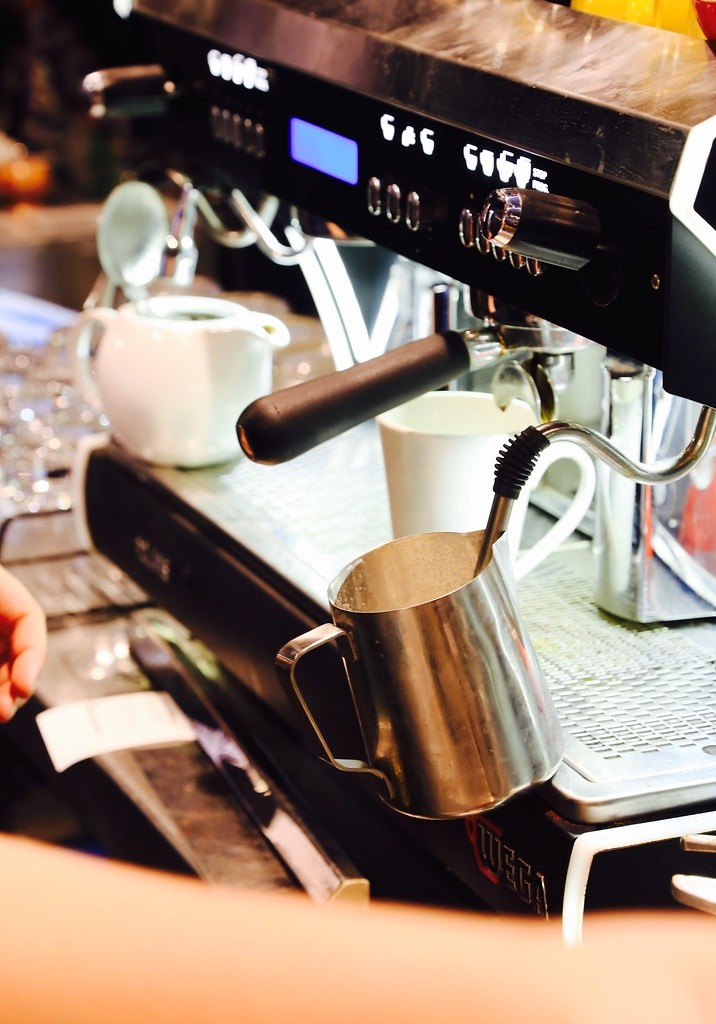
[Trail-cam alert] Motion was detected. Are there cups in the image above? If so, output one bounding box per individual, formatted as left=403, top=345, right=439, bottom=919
left=375, top=390, right=596, bottom=582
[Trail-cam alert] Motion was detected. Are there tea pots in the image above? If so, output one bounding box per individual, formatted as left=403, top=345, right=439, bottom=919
left=275, top=531, right=564, bottom=825
left=67, top=294, right=291, bottom=469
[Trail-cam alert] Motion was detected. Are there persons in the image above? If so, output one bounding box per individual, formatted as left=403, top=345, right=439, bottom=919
left=0, top=564, right=716, bottom=1024
